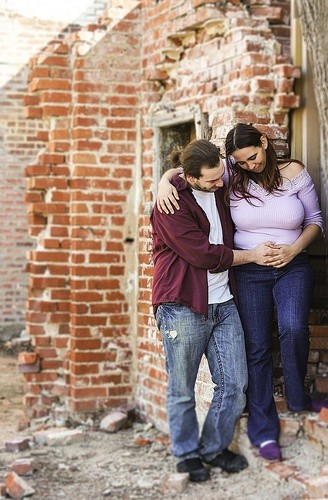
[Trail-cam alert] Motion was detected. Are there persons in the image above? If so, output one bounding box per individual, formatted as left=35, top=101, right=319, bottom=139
left=157, top=122, right=328, bottom=460
left=150, top=137, right=281, bottom=483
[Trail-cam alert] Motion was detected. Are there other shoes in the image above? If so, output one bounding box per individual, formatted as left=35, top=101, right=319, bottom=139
left=176, top=458, right=209, bottom=482
left=259, top=440, right=281, bottom=462
left=211, top=447, right=247, bottom=472
left=309, top=397, right=328, bottom=413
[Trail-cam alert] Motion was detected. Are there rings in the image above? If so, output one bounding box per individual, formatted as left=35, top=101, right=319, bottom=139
left=280, top=260, right=283, bottom=264
